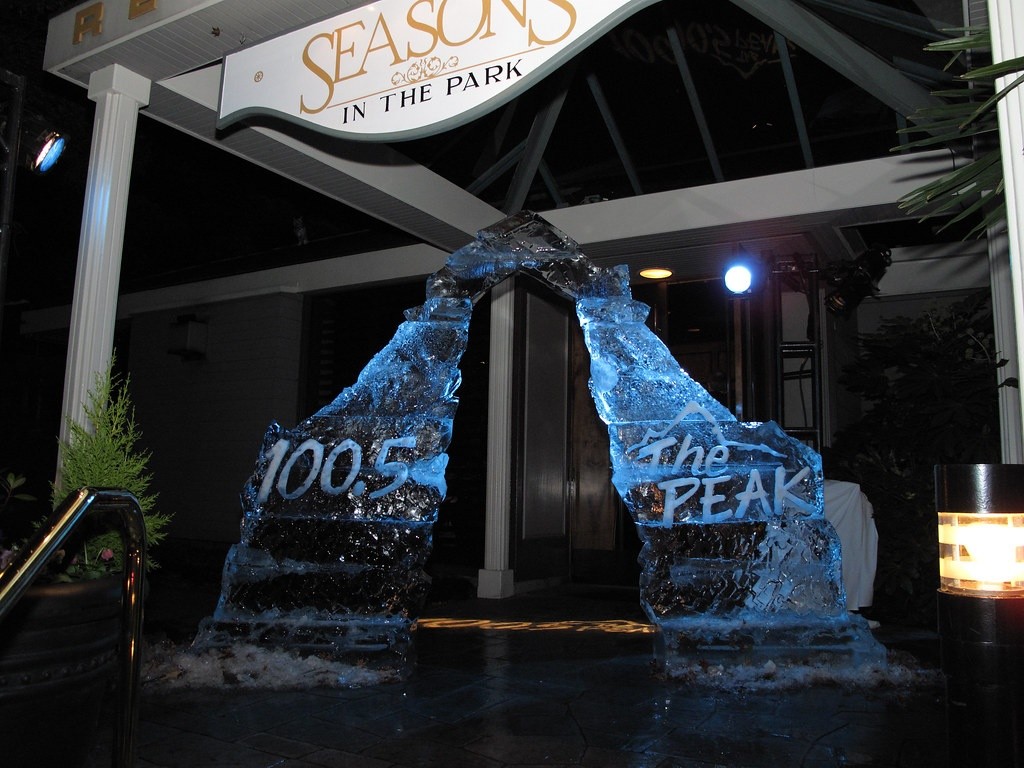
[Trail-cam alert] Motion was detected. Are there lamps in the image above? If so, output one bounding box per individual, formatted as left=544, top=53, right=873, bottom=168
left=828, top=265, right=874, bottom=312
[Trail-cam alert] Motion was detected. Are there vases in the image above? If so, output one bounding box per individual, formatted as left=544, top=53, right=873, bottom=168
left=0, top=574, right=124, bottom=768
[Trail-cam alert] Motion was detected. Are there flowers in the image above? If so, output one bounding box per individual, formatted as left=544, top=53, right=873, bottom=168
left=0, top=347, right=175, bottom=585
